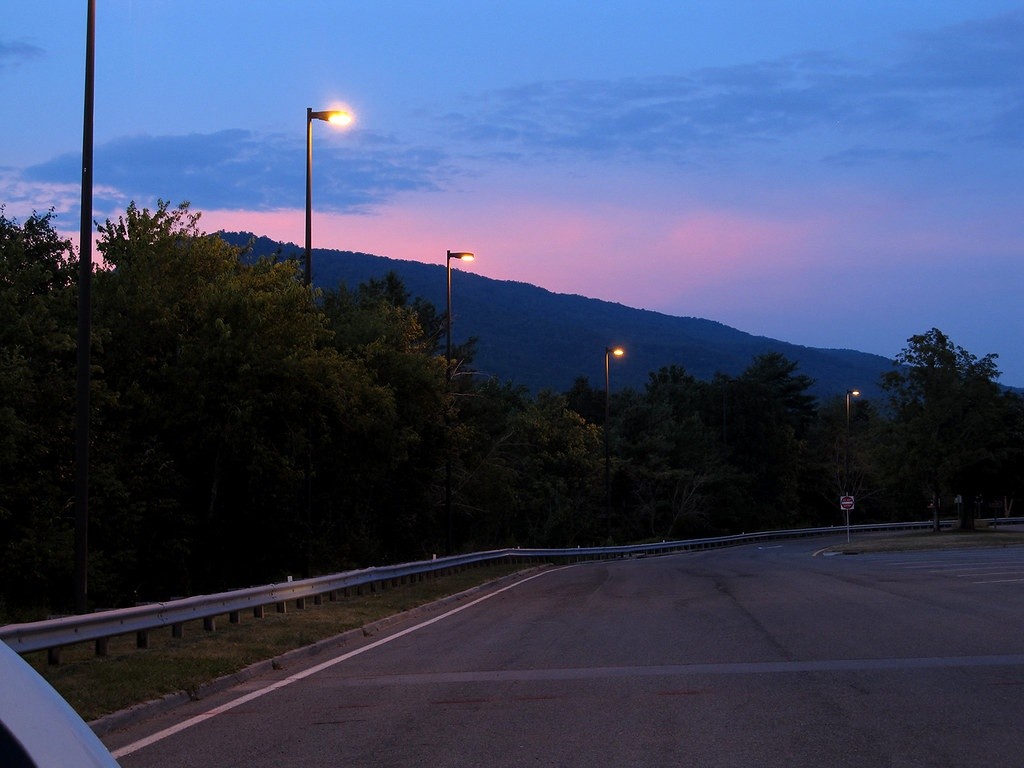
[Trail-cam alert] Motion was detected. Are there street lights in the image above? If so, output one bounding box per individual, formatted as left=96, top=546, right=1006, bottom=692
left=301, top=108, right=365, bottom=560
left=845, top=389, right=860, bottom=496
left=604, top=345, right=625, bottom=547
left=445, top=250, right=477, bottom=556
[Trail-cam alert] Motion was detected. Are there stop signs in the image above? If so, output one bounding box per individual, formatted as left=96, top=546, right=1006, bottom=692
left=840, top=495, right=855, bottom=511
